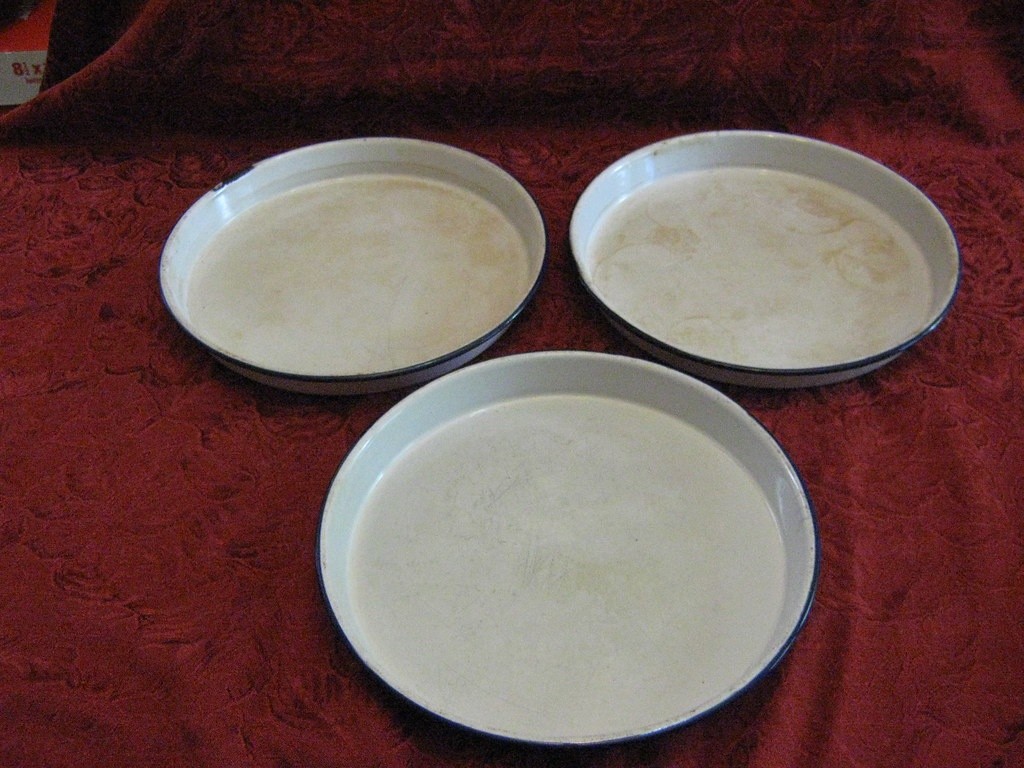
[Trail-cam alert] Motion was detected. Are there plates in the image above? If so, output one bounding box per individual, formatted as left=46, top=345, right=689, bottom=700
left=161, top=134, right=548, bottom=396
left=315, top=351, right=818, bottom=746
left=569, top=129, right=960, bottom=389
